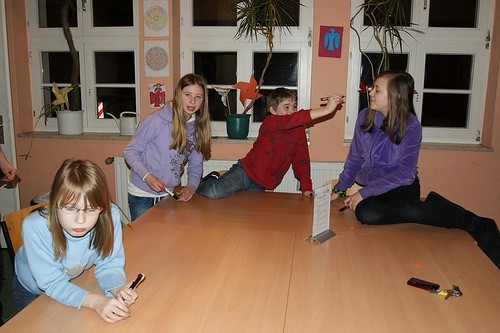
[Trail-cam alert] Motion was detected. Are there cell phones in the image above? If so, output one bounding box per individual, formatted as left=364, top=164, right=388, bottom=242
left=407, top=278, right=440, bottom=291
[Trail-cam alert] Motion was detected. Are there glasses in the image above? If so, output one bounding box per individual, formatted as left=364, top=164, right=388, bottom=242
left=55, top=203, right=102, bottom=216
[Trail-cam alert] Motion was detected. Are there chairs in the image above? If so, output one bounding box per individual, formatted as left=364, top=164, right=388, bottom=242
left=2, top=201, right=52, bottom=267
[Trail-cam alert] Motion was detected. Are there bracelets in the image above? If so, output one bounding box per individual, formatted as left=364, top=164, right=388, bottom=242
left=332, top=187, right=347, bottom=197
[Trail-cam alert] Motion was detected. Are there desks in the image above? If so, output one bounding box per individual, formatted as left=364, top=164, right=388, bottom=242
left=0, top=187, right=500, bottom=333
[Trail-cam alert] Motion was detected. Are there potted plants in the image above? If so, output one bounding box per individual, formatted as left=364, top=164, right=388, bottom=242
left=224, top=0, right=309, bottom=140
left=19, top=0, right=84, bottom=162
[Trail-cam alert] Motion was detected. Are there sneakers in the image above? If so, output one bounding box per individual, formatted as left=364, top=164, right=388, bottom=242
left=200, top=171, right=221, bottom=180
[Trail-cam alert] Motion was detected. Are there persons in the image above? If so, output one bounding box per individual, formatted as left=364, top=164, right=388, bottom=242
left=329, top=70, right=497, bottom=243
left=196, top=88, right=345, bottom=200
left=123, top=73, right=212, bottom=222
left=13, top=158, right=138, bottom=324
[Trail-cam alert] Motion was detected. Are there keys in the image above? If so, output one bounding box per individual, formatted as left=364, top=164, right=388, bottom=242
left=444, top=290, right=454, bottom=300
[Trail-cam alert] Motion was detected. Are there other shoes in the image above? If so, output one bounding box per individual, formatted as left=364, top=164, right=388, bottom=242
left=472, top=215, right=500, bottom=266
left=425, top=190, right=465, bottom=209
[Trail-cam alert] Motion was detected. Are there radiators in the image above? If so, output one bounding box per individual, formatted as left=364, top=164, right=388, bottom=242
left=103, top=156, right=346, bottom=225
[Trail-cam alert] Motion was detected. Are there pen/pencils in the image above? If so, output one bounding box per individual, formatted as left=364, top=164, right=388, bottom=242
left=339, top=205, right=350, bottom=212
left=164, top=188, right=178, bottom=200
left=321, top=96, right=346, bottom=101
left=122, top=273, right=145, bottom=301
